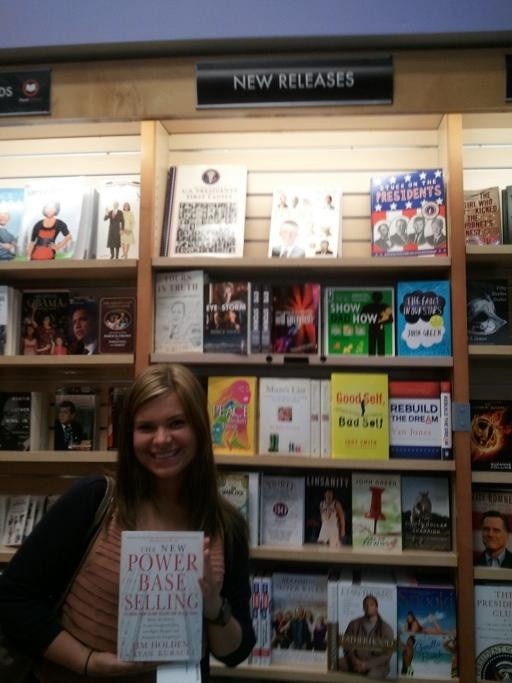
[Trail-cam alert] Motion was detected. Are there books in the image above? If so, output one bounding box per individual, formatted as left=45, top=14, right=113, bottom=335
left=2, top=174, right=146, bottom=563
left=151, top=267, right=512, bottom=683
left=118, top=530, right=204, bottom=663
left=152, top=157, right=512, bottom=259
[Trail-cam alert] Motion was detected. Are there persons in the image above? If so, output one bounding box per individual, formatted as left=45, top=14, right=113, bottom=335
left=2, top=360, right=257, bottom=683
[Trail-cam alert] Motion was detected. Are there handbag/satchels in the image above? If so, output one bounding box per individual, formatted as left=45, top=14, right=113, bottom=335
left=0, top=637, right=32, bottom=681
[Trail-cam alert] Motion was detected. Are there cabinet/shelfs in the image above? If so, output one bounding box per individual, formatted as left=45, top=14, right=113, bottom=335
left=0, top=112, right=511, bottom=680
